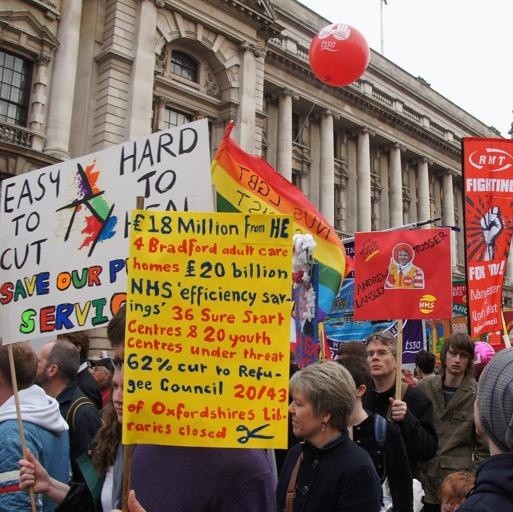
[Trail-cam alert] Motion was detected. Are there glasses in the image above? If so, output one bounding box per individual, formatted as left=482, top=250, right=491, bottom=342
left=367, top=350, right=397, bottom=356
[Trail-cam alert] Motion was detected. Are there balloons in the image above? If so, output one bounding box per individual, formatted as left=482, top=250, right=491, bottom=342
left=307, top=21, right=371, bottom=88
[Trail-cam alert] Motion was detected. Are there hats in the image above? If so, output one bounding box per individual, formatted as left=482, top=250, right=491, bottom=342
left=89, top=358, right=115, bottom=373
left=476, top=346, right=513, bottom=455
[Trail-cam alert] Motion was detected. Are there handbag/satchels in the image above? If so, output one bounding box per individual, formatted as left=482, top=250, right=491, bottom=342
left=380, top=472, right=426, bottom=511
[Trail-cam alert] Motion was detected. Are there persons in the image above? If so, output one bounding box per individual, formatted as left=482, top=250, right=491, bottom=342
left=479, top=205, right=505, bottom=261
left=381, top=241, right=425, bottom=290
left=2, top=302, right=513, bottom=511
